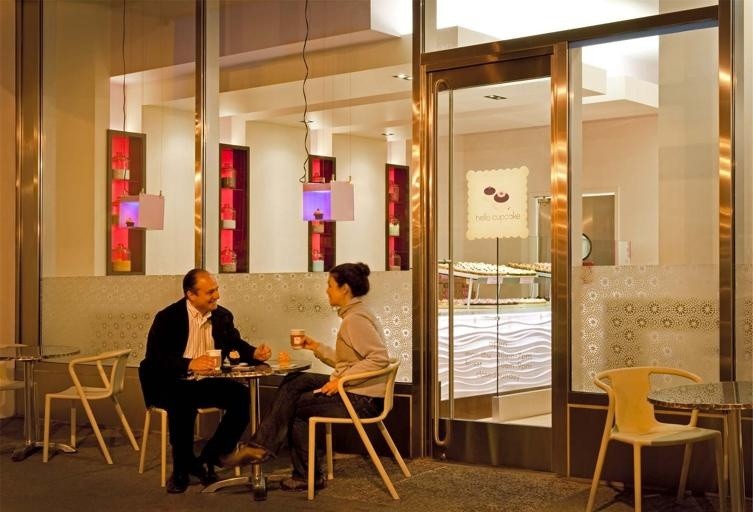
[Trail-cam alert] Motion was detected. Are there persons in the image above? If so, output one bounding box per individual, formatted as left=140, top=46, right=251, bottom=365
left=138, top=267, right=272, bottom=495
left=221, top=260, right=389, bottom=491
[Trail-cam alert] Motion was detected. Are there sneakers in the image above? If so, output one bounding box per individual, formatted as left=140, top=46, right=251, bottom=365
left=279, top=475, right=324, bottom=491
left=166, top=468, right=190, bottom=494
left=190, top=455, right=220, bottom=484
left=221, top=440, right=272, bottom=466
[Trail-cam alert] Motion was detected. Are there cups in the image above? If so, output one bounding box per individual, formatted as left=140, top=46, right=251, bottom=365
left=205, top=349, right=222, bottom=372
left=290, top=328, right=305, bottom=350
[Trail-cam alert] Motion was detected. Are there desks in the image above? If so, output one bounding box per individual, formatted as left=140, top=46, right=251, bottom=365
left=647, top=380, right=753, bottom=512
left=0, top=345, right=80, bottom=462
left=195, top=359, right=312, bottom=500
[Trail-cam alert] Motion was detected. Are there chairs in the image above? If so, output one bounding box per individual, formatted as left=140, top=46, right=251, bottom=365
left=138, top=408, right=241, bottom=487
left=43, top=349, right=140, bottom=464
left=0, top=344, right=39, bottom=440
left=586, top=367, right=727, bottom=512
left=308, top=358, right=412, bottom=499
